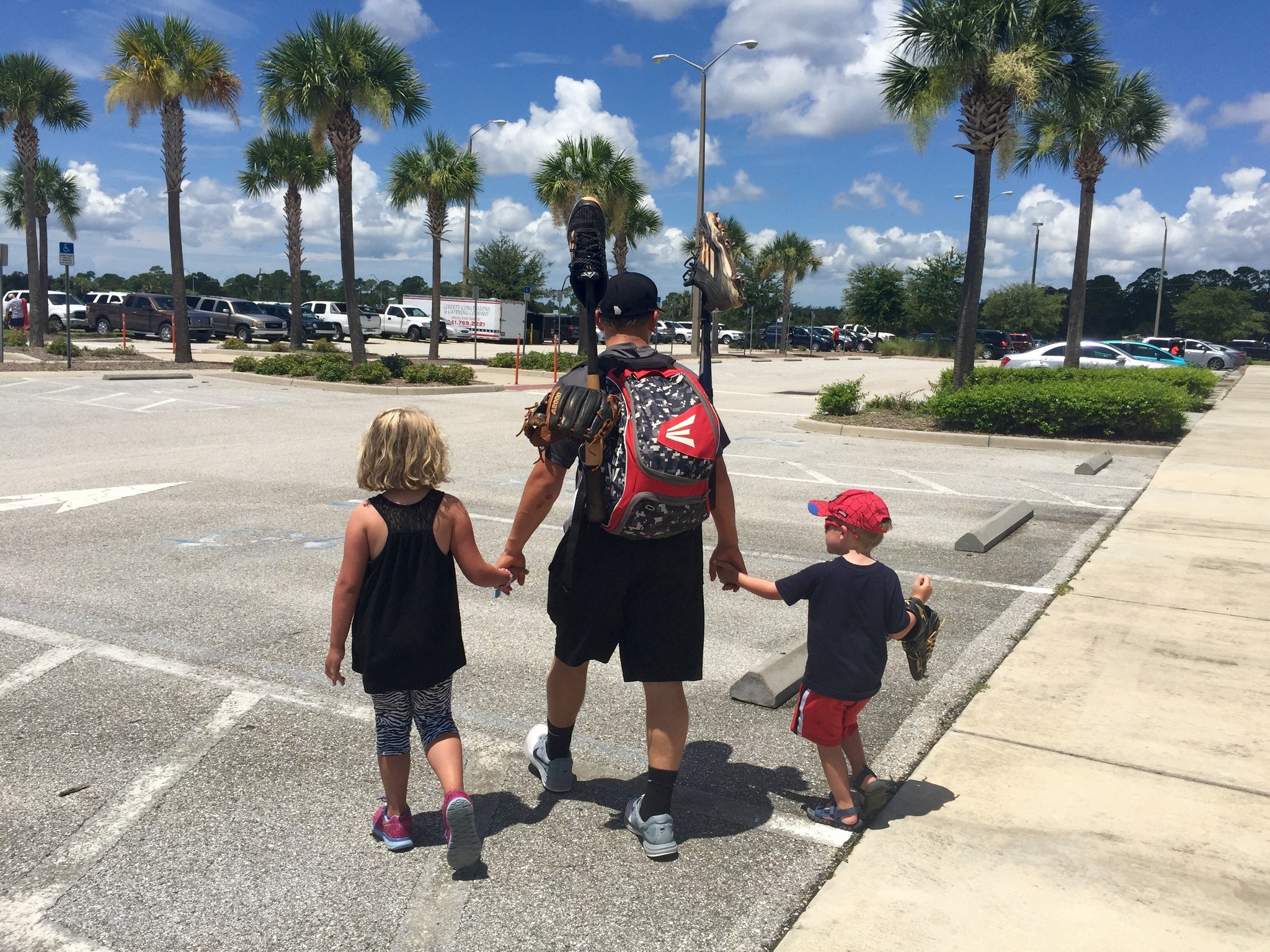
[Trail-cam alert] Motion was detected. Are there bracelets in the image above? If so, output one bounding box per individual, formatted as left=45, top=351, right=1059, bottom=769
left=494, top=568, right=513, bottom=598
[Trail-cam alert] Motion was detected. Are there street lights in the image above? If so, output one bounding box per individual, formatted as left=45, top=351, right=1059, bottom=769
left=184, top=267, right=194, bottom=294
left=1153, top=215, right=1169, bottom=337
left=369, top=273, right=383, bottom=306
left=459, top=119, right=507, bottom=297
left=1032, top=220, right=1043, bottom=283
left=653, top=40, right=755, bottom=353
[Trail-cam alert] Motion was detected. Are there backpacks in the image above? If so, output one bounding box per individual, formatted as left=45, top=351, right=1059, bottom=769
left=572, top=352, right=720, bottom=539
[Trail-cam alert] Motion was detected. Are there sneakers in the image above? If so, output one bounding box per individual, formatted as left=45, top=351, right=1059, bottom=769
left=523, top=723, right=573, bottom=792
left=441, top=788, right=481, bottom=868
left=371, top=796, right=413, bottom=851
left=623, top=794, right=678, bottom=858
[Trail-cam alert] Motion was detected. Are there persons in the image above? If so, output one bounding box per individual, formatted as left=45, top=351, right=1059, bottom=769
left=832, top=327, right=840, bottom=352
left=324, top=408, right=529, bottom=868
left=714, top=489, right=933, bottom=832
left=498, top=271, right=748, bottom=857
left=1171, top=343, right=1179, bottom=355
left=5, top=294, right=24, bottom=335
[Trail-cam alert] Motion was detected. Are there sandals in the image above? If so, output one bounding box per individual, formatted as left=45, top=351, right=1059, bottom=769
left=806, top=796, right=863, bottom=830
left=853, top=765, right=888, bottom=813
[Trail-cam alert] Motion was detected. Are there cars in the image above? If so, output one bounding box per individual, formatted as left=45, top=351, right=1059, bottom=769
left=1001, top=340, right=1181, bottom=369
left=1084, top=341, right=1202, bottom=369
left=539, top=312, right=1047, bottom=358
left=429, top=315, right=472, bottom=342
left=1202, top=340, right=1270, bottom=366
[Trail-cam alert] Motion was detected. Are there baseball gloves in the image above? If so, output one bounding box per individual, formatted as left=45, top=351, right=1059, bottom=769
left=901, top=596, right=940, bottom=681
left=524, top=383, right=618, bottom=449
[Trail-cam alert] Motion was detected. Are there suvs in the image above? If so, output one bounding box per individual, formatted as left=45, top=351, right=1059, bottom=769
left=84, top=291, right=337, bottom=343
left=1143, top=336, right=1234, bottom=370
left=2, top=289, right=96, bottom=333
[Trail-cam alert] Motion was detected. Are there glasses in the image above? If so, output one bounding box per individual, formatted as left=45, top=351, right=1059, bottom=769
left=825, top=518, right=858, bottom=539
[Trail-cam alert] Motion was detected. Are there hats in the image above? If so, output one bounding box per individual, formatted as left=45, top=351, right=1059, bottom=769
left=598, top=272, right=665, bottom=317
left=808, top=489, right=891, bottom=533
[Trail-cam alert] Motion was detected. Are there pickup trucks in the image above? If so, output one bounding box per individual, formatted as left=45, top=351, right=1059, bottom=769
left=86, top=294, right=214, bottom=343
left=301, top=302, right=382, bottom=343
left=377, top=304, right=447, bottom=343
